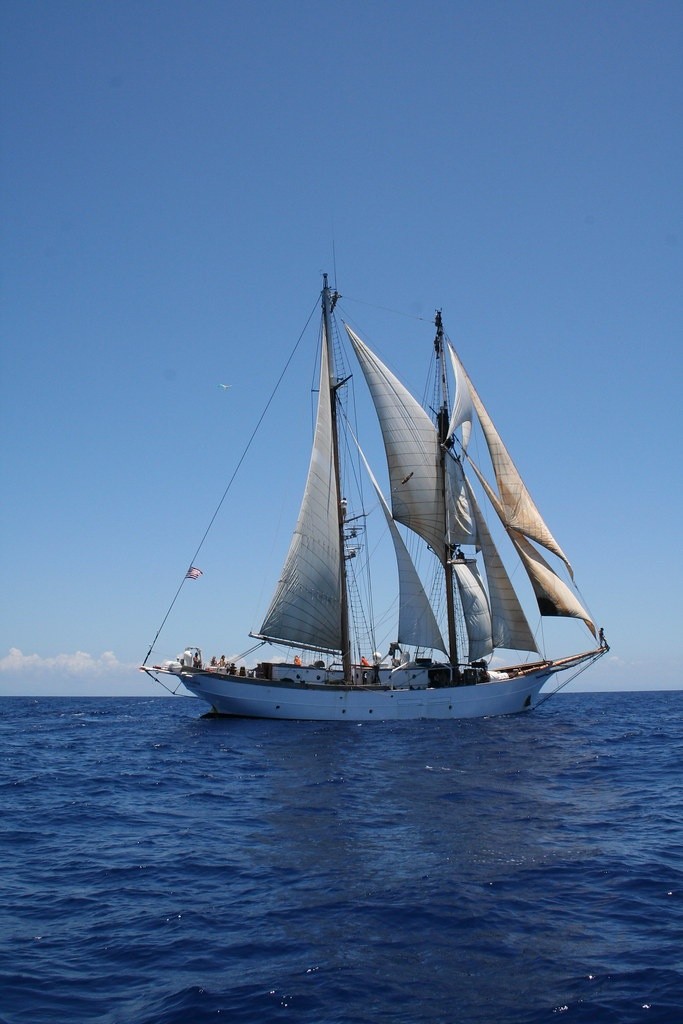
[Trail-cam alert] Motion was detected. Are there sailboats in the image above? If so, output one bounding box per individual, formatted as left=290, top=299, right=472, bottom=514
left=138, top=270, right=615, bottom=725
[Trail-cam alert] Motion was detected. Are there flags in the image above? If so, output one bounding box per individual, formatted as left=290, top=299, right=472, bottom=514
left=185, top=567, right=203, bottom=580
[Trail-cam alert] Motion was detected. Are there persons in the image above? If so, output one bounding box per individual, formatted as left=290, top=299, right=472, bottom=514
left=362, top=656, right=369, bottom=666
left=339, top=498, right=347, bottom=522
left=294, top=655, right=302, bottom=665
left=220, top=655, right=230, bottom=668
left=211, top=656, right=216, bottom=666
left=457, top=549, right=464, bottom=559
left=193, top=652, right=198, bottom=667
